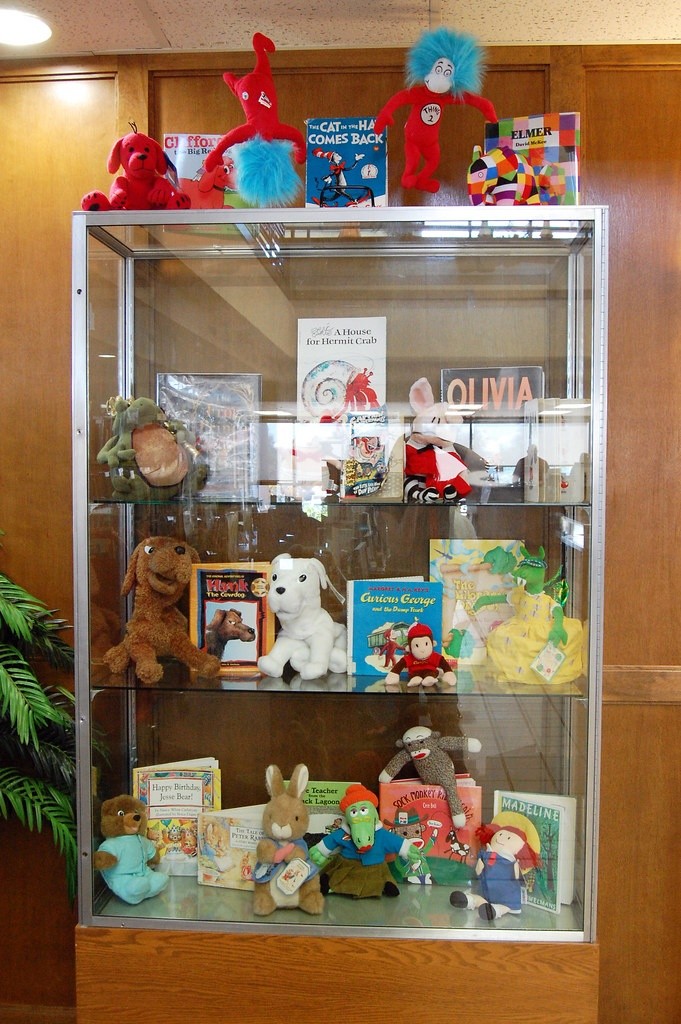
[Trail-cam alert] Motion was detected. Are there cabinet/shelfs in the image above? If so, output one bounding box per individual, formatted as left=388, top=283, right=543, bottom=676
left=68, top=203, right=600, bottom=1024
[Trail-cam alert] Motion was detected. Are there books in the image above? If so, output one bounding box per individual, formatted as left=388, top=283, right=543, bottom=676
left=163, top=108, right=581, bottom=238
left=186, top=539, right=526, bottom=671
left=131, top=755, right=579, bottom=913
left=155, top=316, right=585, bottom=504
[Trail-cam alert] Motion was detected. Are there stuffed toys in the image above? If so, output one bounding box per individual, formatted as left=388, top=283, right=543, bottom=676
left=97, top=394, right=209, bottom=500
left=379, top=727, right=482, bottom=828
left=103, top=537, right=220, bottom=681
left=385, top=620, right=458, bottom=686
left=251, top=762, right=324, bottom=917
left=257, top=553, right=347, bottom=679
left=402, top=377, right=474, bottom=499
left=93, top=795, right=170, bottom=906
left=203, top=33, right=305, bottom=207
left=472, top=545, right=583, bottom=685
left=81, top=122, right=192, bottom=211
left=373, top=25, right=500, bottom=193
left=308, top=785, right=423, bottom=897
left=449, top=809, right=542, bottom=920
